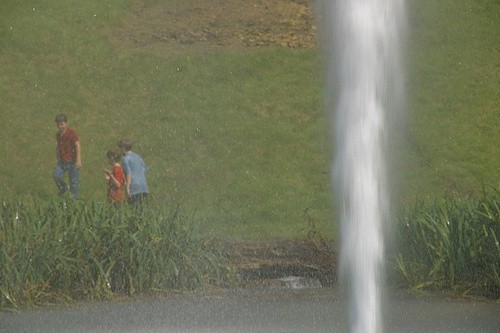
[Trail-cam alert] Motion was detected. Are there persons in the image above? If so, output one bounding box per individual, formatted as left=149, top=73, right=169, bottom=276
left=103, top=148, right=125, bottom=204
left=118, top=139, right=150, bottom=205
left=53, top=113, right=82, bottom=197
